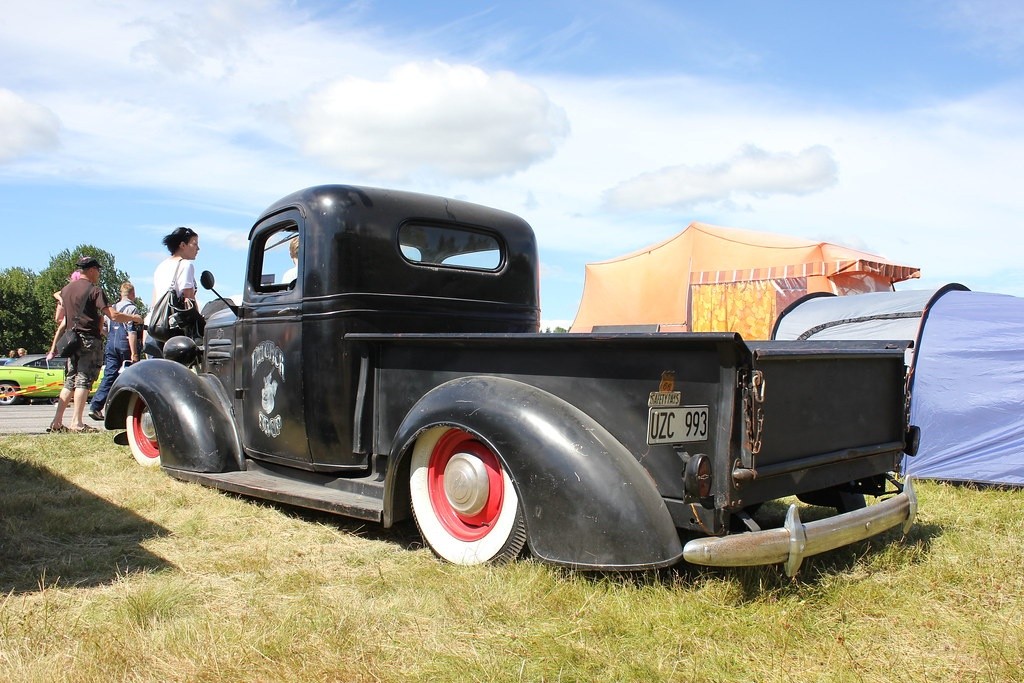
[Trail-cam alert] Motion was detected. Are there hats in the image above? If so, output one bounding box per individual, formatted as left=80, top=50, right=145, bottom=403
left=76, top=256, right=104, bottom=269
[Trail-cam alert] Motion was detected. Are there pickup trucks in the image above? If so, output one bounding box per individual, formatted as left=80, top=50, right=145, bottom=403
left=103, top=184, right=923, bottom=578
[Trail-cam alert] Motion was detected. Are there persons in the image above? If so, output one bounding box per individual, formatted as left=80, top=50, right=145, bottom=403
left=147, top=227, right=201, bottom=367
left=47, top=257, right=144, bottom=433
left=18, top=348, right=25, bottom=357
left=10, top=350, right=17, bottom=358
left=283, top=236, right=299, bottom=283
left=89, top=283, right=140, bottom=420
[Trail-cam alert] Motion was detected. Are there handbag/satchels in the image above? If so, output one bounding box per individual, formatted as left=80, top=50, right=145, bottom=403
left=56, top=330, right=83, bottom=358
left=147, top=258, right=196, bottom=342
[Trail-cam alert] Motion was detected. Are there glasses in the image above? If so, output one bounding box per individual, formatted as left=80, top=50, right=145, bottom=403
left=182, top=229, right=193, bottom=241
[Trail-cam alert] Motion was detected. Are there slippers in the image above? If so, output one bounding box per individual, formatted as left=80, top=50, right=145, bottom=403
left=70, top=424, right=98, bottom=433
left=46, top=425, right=69, bottom=433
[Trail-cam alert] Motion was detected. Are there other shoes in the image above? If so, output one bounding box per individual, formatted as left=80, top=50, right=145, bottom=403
left=89, top=409, right=105, bottom=421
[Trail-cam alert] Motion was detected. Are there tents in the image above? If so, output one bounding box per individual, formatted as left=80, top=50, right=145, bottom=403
left=569, top=222, right=921, bottom=343
left=771, top=282, right=1024, bottom=486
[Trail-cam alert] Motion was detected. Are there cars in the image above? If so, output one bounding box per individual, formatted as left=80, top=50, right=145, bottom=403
left=0, top=353, right=106, bottom=406
